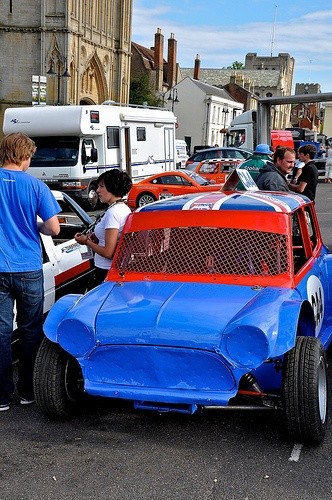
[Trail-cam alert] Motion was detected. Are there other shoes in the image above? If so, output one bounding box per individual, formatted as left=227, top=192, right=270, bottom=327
left=0, top=396, right=15, bottom=411
left=20, top=394, right=36, bottom=404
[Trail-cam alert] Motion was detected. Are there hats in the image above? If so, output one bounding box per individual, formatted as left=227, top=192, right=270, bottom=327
left=253, top=144, right=272, bottom=154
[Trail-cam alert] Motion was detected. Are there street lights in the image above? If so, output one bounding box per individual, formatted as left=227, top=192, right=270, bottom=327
left=222, top=102, right=230, bottom=128
left=168, top=84, right=180, bottom=111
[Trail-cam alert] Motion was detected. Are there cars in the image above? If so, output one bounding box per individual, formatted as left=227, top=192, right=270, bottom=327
left=175, top=140, right=254, bottom=183
left=127, top=170, right=224, bottom=207
left=13, top=190, right=97, bottom=329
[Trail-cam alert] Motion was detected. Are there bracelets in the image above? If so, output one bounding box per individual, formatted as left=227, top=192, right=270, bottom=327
left=83, top=238, right=90, bottom=245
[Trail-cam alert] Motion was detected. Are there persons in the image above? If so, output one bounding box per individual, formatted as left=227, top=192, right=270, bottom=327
left=255, top=147, right=296, bottom=192
left=320, top=136, right=332, bottom=184
left=268, top=145, right=274, bottom=158
left=0, top=131, right=64, bottom=412
left=291, top=145, right=305, bottom=184
left=235, top=143, right=276, bottom=191
left=72, top=168, right=133, bottom=289
left=287, top=144, right=319, bottom=206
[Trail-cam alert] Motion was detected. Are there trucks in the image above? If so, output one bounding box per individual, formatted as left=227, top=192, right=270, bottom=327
left=223, top=93, right=332, bottom=179
left=3, top=99, right=177, bottom=210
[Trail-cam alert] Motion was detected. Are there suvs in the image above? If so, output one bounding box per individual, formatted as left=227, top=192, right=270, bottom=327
left=34, top=191, right=332, bottom=443
